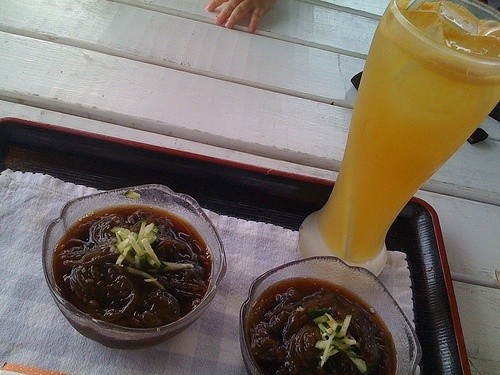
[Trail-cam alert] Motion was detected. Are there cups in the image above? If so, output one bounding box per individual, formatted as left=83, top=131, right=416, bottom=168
left=299, top=0, right=500, bottom=278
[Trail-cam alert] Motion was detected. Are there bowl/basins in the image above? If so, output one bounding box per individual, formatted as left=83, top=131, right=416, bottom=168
left=42, top=185, right=228, bottom=349
left=239, top=256, right=422, bottom=375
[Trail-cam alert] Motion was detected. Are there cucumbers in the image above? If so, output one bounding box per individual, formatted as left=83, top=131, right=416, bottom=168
left=124, top=191, right=140, bottom=199
left=307, top=308, right=368, bottom=374
left=108, top=221, right=194, bottom=291
